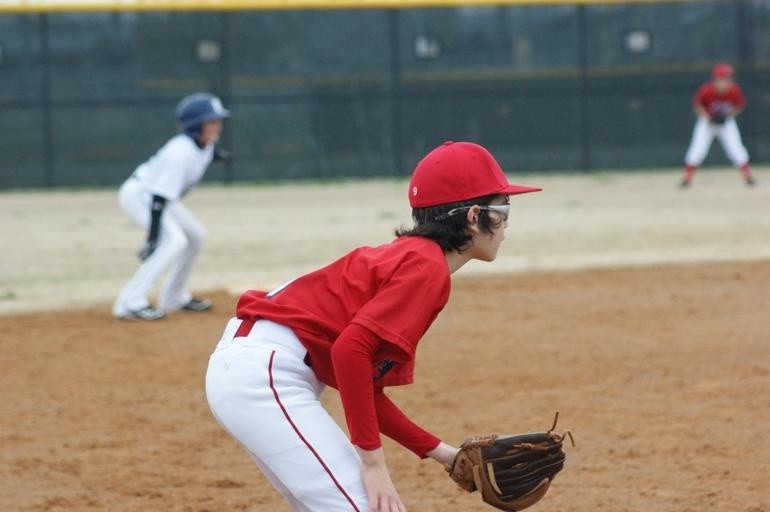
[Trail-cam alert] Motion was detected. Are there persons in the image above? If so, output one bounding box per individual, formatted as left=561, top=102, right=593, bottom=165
left=677, top=62, right=757, bottom=190
left=111, top=93, right=233, bottom=323
left=204, top=140, right=546, bottom=512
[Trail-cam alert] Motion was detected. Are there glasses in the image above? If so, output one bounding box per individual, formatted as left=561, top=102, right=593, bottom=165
left=435, top=200, right=511, bottom=221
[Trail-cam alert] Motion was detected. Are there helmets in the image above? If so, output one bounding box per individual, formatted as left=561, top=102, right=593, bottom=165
left=176, top=91, right=229, bottom=132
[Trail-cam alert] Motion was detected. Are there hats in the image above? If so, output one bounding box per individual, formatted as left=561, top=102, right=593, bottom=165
left=715, top=61, right=732, bottom=77
left=409, top=141, right=542, bottom=207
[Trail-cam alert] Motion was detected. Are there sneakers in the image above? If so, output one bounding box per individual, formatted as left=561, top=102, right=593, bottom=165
left=119, top=295, right=213, bottom=320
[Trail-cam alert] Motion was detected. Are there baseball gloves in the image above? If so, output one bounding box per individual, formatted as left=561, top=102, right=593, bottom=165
left=445, top=412, right=575, bottom=512
left=710, top=101, right=729, bottom=124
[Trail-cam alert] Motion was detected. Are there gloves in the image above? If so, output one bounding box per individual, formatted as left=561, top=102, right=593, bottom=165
left=139, top=244, right=158, bottom=260
